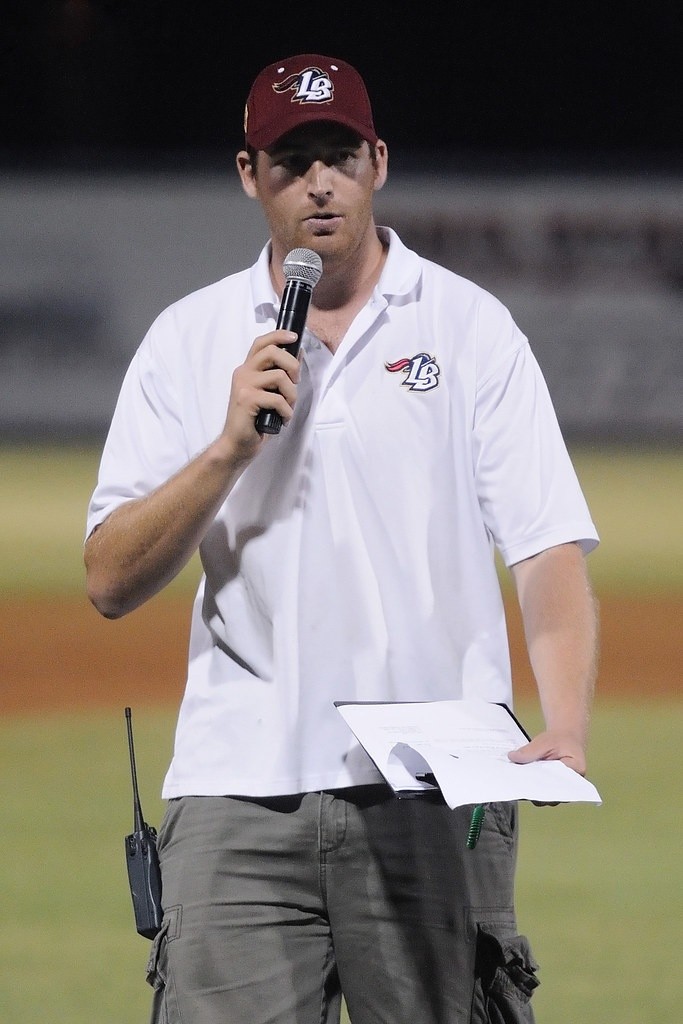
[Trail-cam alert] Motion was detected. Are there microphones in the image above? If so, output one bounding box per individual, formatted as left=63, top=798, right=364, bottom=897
left=253, top=248, right=322, bottom=434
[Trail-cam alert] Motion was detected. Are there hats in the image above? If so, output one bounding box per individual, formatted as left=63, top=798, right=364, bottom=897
left=243, top=54, right=378, bottom=151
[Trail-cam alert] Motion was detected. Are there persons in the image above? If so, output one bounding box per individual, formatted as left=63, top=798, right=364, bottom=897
left=82, top=54, right=600, bottom=1024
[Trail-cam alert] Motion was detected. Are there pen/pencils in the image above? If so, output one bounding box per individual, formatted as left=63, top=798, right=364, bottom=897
left=414, top=770, right=439, bottom=785
